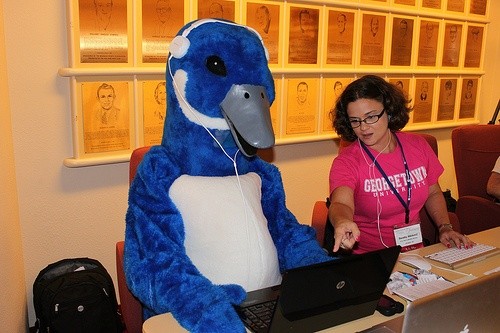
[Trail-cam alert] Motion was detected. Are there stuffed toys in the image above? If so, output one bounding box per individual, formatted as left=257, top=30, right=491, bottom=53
left=122, top=17, right=333, bottom=333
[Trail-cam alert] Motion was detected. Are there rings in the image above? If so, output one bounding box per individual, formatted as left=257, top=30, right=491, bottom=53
left=340, top=243, right=343, bottom=246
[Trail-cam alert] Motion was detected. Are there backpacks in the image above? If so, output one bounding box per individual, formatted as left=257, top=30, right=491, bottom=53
left=33, top=257, right=126, bottom=333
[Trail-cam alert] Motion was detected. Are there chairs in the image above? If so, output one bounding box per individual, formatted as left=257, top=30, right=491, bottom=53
left=310, top=134, right=459, bottom=248
left=451, top=125, right=500, bottom=235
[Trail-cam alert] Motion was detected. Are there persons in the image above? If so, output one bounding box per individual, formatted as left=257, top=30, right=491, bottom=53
left=329, top=75, right=477, bottom=258
left=487, top=156, right=500, bottom=198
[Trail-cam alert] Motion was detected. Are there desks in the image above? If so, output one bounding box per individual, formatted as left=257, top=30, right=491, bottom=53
left=143, top=226, right=500, bottom=333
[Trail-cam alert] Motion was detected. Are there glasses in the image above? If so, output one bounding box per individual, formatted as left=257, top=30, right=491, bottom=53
left=349, top=105, right=388, bottom=128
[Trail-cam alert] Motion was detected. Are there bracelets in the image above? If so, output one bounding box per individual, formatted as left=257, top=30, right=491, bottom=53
left=439, top=224, right=452, bottom=231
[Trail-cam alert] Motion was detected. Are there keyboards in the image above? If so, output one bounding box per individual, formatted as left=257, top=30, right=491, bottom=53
left=422, top=241, right=500, bottom=271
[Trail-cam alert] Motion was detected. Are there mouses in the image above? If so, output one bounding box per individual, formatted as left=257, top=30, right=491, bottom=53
left=399, top=257, right=431, bottom=271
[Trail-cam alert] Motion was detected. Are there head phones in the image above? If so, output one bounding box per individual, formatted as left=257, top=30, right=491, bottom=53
left=169, top=18, right=270, bottom=60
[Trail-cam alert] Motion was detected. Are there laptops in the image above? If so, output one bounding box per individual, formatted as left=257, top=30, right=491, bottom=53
left=234, top=246, right=401, bottom=333
